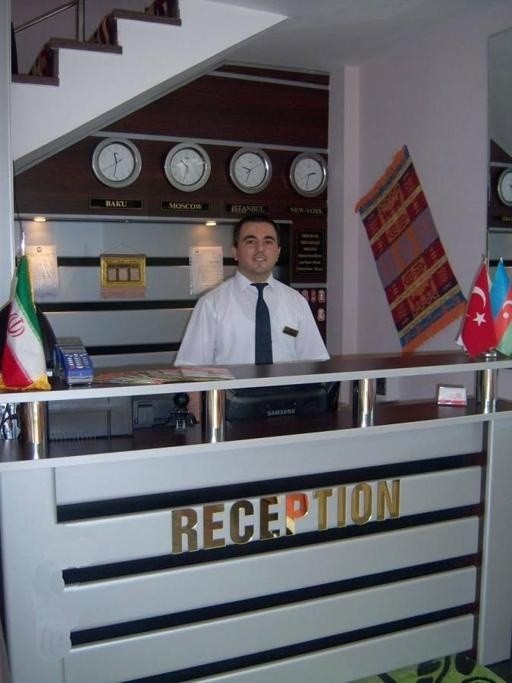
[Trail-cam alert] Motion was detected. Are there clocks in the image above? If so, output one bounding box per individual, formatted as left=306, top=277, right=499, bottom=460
left=164, top=142, right=211, bottom=192
left=91, top=137, right=142, bottom=189
left=497, top=168, right=512, bottom=207
left=289, top=153, right=329, bottom=198
left=229, top=147, right=273, bottom=195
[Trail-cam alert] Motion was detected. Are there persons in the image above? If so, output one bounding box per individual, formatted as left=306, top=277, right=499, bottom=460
left=172, top=212, right=332, bottom=366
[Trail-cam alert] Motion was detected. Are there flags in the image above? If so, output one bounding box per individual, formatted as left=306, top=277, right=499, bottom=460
left=453, top=254, right=497, bottom=356
left=489, top=260, right=512, bottom=358
left=2, top=241, right=54, bottom=394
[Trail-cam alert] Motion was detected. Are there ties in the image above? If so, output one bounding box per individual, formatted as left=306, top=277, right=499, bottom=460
left=251, top=283, right=272, bottom=366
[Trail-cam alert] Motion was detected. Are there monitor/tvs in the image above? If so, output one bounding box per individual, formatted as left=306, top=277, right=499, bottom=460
left=200, top=382, right=339, bottom=430
